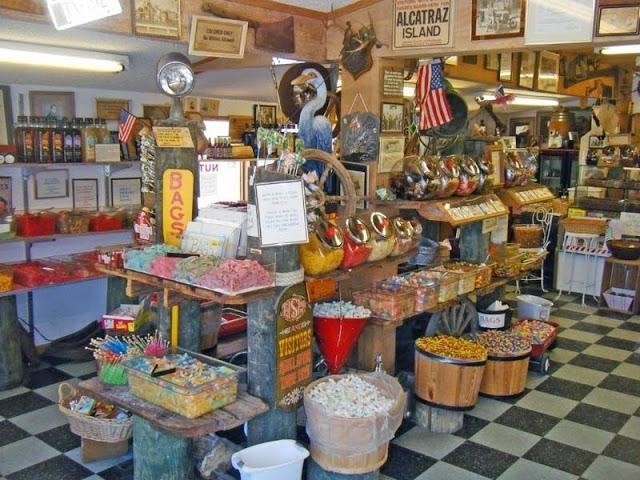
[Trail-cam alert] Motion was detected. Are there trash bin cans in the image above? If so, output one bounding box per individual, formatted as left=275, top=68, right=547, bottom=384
left=231, top=438, right=310, bottom=480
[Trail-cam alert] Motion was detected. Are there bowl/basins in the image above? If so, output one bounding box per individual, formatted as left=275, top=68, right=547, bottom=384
left=512, top=224, right=544, bottom=248
left=606, top=240, right=640, bottom=260
left=563, top=139, right=574, bottom=149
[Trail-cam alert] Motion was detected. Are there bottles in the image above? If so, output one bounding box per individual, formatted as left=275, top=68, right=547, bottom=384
left=479, top=119, right=487, bottom=136
left=243, top=123, right=299, bottom=159
left=205, top=138, right=233, bottom=159
left=14, top=115, right=112, bottom=164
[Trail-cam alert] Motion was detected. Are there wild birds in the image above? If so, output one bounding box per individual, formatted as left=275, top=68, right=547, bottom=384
left=289, top=67, right=334, bottom=194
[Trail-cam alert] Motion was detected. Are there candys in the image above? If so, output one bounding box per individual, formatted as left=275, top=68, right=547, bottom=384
left=77, top=127, right=555, bottom=420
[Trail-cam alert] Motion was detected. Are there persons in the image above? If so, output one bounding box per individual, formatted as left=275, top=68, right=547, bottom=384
left=45, top=104, right=58, bottom=121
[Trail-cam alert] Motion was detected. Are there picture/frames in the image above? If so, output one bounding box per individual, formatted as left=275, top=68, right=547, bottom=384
left=485, top=53, right=499, bottom=71
left=462, top=54, right=478, bottom=65
left=443, top=55, right=459, bottom=67
left=379, top=100, right=406, bottom=135
left=590, top=0, right=639, bottom=38
left=390, top=0, right=456, bottom=50
left=377, top=135, right=406, bottom=174
left=517, top=50, right=537, bottom=92
left=537, top=49, right=561, bottom=94
left=0, top=83, right=279, bottom=221
left=186, top=13, right=250, bottom=61
left=499, top=52, right=514, bottom=83
left=129, top=1, right=182, bottom=41
left=468, top=0, right=528, bottom=39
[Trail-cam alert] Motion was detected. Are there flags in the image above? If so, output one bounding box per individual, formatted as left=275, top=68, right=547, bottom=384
left=415, top=61, right=454, bottom=130
left=118, top=108, right=136, bottom=144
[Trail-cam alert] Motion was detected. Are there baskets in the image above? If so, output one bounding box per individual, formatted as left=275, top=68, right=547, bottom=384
left=57, top=381, right=134, bottom=444
left=560, top=217, right=608, bottom=235
left=512, top=226, right=543, bottom=249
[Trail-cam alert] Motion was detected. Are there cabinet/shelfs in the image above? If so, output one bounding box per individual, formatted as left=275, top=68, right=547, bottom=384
left=77, top=258, right=276, bottom=480
left=0, top=225, right=139, bottom=370
left=536, top=148, right=579, bottom=190
left=367, top=201, right=556, bottom=333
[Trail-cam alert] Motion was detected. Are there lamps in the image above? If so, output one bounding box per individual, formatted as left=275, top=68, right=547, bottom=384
left=153, top=51, right=198, bottom=126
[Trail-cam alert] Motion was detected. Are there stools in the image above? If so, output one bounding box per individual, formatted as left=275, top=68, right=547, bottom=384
left=600, top=258, right=640, bottom=320
left=553, top=231, right=600, bottom=307
